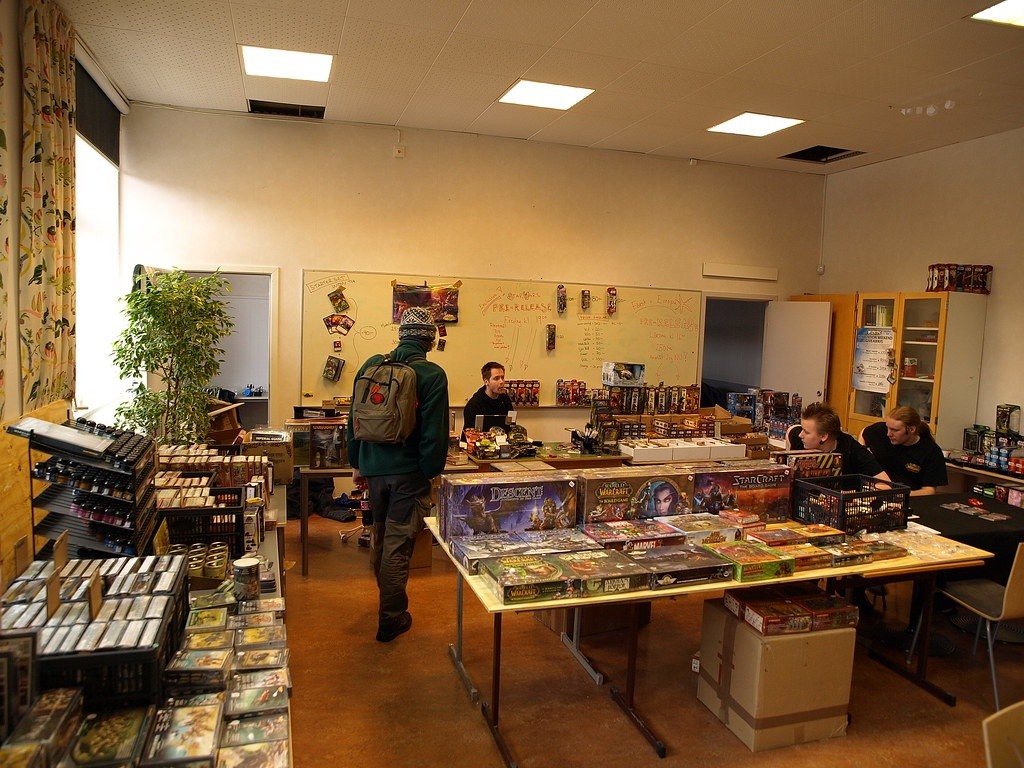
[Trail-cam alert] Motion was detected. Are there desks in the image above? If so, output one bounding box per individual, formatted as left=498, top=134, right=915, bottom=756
left=904, top=495, right=1024, bottom=653
left=300, top=459, right=478, bottom=575
left=459, top=442, right=631, bottom=472
left=425, top=516, right=995, bottom=767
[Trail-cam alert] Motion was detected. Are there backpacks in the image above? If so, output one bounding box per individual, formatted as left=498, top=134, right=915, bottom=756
left=352, top=354, right=430, bottom=444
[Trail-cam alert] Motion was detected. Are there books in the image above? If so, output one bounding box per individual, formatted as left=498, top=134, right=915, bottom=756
left=444, top=459, right=479, bottom=470
left=904, top=357, right=917, bottom=378
left=864, top=304, right=893, bottom=327
left=916, top=334, right=937, bottom=342
left=940, top=502, right=1009, bottom=522
left=322, top=355, right=345, bottom=382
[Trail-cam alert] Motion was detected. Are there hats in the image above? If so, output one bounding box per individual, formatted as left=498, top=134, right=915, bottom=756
left=399, top=306, right=436, bottom=347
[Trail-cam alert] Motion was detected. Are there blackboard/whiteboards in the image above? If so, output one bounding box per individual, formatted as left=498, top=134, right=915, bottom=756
left=298, top=268, right=702, bottom=408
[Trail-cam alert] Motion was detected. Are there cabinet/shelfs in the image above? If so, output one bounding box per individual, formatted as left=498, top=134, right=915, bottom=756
left=28, top=408, right=157, bottom=560
left=847, top=291, right=987, bottom=449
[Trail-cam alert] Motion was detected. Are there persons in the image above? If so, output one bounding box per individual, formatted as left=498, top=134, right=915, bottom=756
left=858, top=406, right=949, bottom=496
left=460, top=362, right=517, bottom=442
left=347, top=307, right=450, bottom=641
left=790, top=403, right=893, bottom=490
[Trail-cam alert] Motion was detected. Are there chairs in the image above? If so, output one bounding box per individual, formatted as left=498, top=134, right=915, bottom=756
left=904, top=542, right=1022, bottom=712
left=982, top=700, right=1024, bottom=767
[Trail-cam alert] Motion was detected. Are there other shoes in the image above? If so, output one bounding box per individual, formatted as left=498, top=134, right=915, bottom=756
left=376, top=611, right=412, bottom=642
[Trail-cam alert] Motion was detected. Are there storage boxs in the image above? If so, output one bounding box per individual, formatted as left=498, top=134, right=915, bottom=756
left=765, top=580, right=860, bottom=632
left=698, top=597, right=856, bottom=751
left=433, top=362, right=911, bottom=605
left=409, top=527, right=432, bottom=569
left=924, top=263, right=993, bottom=295
left=942, top=403, right=1024, bottom=508
left=0, top=554, right=192, bottom=716
left=532, top=596, right=651, bottom=638
left=903, top=358, right=917, bottom=377
left=724, top=585, right=811, bottom=635
left=155, top=395, right=351, bottom=559
